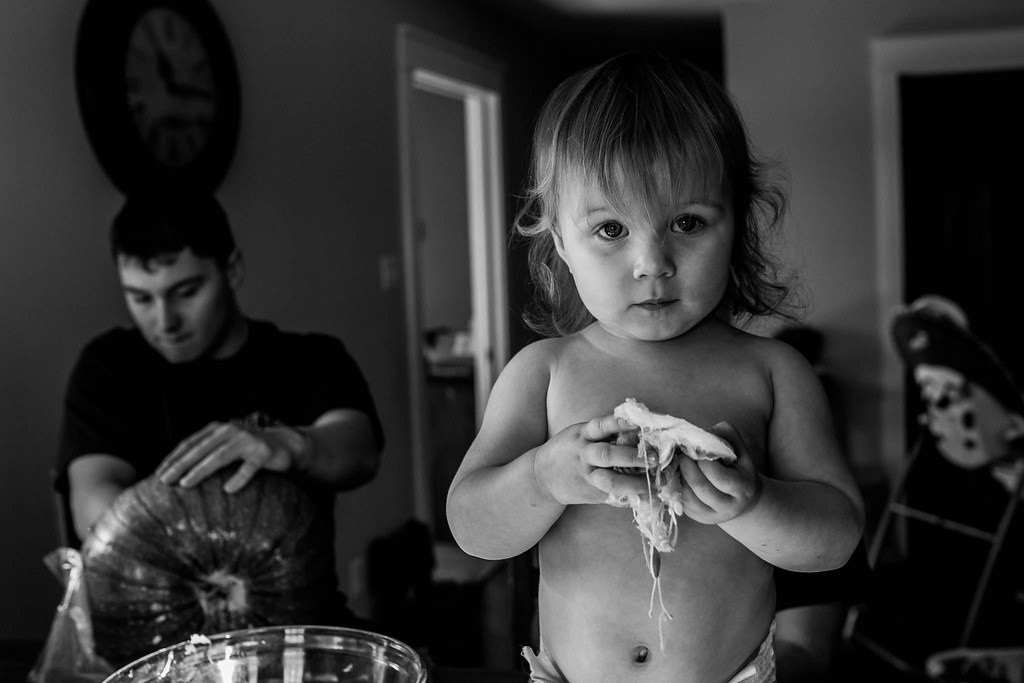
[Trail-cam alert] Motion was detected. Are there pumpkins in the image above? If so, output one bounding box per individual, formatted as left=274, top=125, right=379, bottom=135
left=79, top=465, right=325, bottom=674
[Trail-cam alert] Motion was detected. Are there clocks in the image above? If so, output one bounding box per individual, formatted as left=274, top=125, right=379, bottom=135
left=70, top=0, right=242, bottom=206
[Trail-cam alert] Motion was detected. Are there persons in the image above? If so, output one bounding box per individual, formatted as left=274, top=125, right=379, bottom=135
left=54, top=177, right=386, bottom=676
left=447, top=47, right=866, bottom=683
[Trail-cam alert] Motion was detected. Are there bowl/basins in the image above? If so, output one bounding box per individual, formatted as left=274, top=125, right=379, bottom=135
left=100, top=625, right=428, bottom=683
